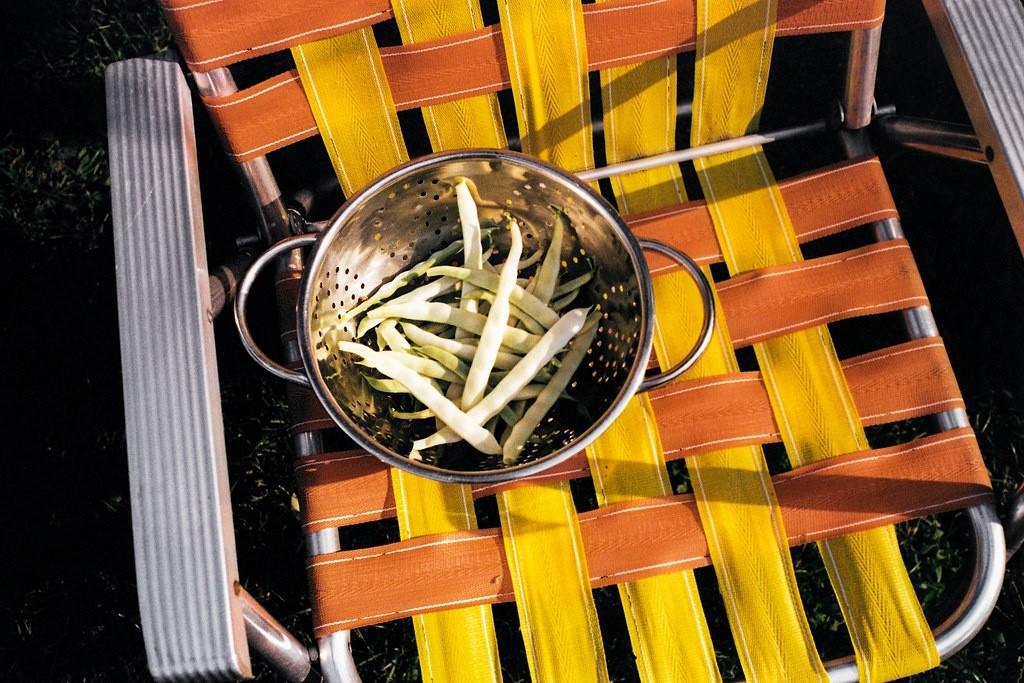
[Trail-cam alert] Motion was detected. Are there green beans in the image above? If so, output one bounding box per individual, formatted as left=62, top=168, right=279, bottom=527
left=333, top=180, right=602, bottom=465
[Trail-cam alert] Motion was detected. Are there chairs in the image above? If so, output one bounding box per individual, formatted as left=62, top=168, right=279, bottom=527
left=107, top=1, right=1024, bottom=683
